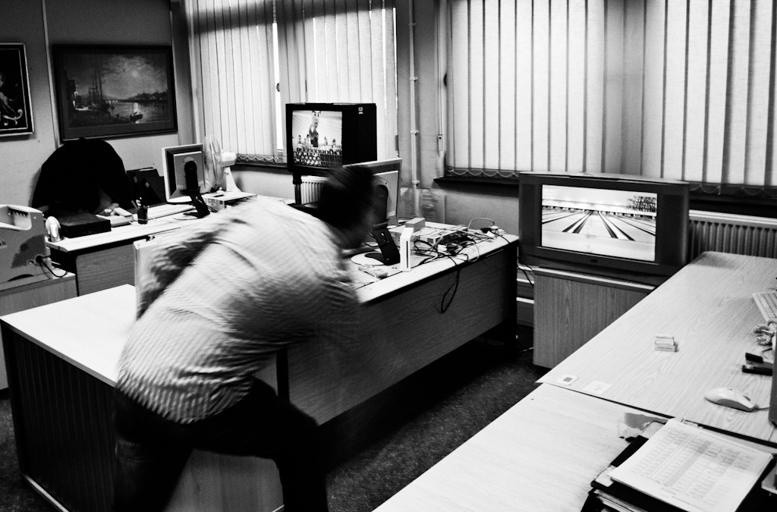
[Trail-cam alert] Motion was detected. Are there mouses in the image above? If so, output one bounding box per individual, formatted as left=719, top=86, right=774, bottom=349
left=704, top=387, right=756, bottom=412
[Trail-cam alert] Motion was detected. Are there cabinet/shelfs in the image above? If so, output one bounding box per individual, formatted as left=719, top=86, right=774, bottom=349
left=1, top=282, right=286, bottom=511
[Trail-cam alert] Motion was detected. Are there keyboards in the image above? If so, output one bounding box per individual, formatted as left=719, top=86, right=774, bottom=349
left=752, top=290, right=776, bottom=322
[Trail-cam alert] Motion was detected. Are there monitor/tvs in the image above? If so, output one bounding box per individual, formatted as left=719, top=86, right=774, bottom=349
left=343, top=158, right=403, bottom=266
left=285, top=103, right=377, bottom=180
left=163, top=143, right=210, bottom=219
left=518, top=173, right=690, bottom=287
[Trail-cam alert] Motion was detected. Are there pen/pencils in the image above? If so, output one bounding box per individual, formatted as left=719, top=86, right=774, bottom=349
left=131, top=197, right=150, bottom=210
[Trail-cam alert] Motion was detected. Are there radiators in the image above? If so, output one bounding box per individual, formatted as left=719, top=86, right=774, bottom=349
left=689, top=210, right=775, bottom=259
left=299, top=175, right=328, bottom=205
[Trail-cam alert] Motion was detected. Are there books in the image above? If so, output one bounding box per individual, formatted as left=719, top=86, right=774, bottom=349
left=588, top=418, right=777, bottom=512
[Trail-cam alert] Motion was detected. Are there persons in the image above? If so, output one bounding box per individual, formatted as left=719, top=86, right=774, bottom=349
left=107, top=166, right=378, bottom=512
left=307, top=111, right=320, bottom=147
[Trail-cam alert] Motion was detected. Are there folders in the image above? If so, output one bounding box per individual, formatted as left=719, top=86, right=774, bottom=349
left=581, top=414, right=776, bottom=512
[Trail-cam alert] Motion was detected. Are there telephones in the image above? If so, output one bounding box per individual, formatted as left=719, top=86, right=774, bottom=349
left=96, top=207, right=132, bottom=227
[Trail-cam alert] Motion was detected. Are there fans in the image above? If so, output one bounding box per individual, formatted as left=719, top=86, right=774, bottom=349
left=203, top=138, right=255, bottom=201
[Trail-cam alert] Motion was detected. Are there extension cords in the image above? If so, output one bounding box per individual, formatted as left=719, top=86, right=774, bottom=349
left=428, top=223, right=469, bottom=241
left=484, top=226, right=504, bottom=238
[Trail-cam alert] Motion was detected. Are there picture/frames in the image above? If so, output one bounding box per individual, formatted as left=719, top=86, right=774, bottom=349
left=0, top=41, right=36, bottom=135
left=50, top=44, right=180, bottom=143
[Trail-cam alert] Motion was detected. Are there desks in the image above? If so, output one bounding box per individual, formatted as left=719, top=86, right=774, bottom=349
left=1, top=266, right=80, bottom=390
left=248, top=214, right=518, bottom=474
left=536, top=251, right=777, bottom=448
left=41, top=190, right=296, bottom=295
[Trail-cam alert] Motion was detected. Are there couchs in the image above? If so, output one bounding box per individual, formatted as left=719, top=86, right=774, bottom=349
left=370, top=384, right=776, bottom=511
